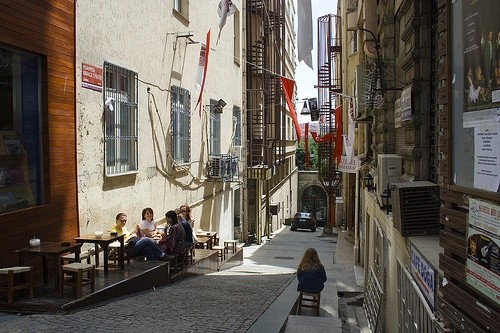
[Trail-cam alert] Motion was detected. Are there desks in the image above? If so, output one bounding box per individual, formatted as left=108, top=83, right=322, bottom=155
left=74, top=231, right=127, bottom=277
left=15, top=242, right=83, bottom=294
left=196, top=231, right=217, bottom=250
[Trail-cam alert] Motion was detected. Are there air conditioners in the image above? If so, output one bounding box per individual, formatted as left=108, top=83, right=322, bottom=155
left=353, top=64, right=368, bottom=117
left=210, top=156, right=237, bottom=178
left=376, top=154, right=402, bottom=209
left=233, top=146, right=243, bottom=161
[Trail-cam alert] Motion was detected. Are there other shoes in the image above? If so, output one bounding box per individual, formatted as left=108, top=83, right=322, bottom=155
left=159, top=254, right=176, bottom=263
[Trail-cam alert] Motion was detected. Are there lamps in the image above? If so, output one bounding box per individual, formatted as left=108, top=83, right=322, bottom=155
left=218, top=98, right=226, bottom=108
left=364, top=170, right=376, bottom=193
left=379, top=183, right=392, bottom=214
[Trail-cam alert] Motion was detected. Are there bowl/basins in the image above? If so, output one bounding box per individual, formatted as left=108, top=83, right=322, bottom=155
left=29, top=238, right=41, bottom=247
left=95, top=231, right=104, bottom=238
left=196, top=229, right=202, bottom=233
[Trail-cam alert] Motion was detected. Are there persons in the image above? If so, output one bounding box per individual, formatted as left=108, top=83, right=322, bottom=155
left=296, top=247, right=327, bottom=294
left=139, top=207, right=157, bottom=236
left=111, top=212, right=175, bottom=261
left=155, top=204, right=199, bottom=258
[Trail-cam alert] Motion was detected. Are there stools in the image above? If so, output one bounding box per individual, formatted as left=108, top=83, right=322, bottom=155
left=0, top=266, right=34, bottom=304
left=298, top=291, right=321, bottom=317
left=59, top=243, right=130, bottom=295
left=212, top=245, right=226, bottom=262
left=224, top=239, right=238, bottom=255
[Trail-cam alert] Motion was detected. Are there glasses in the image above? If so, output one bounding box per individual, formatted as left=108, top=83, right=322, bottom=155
left=182, top=210, right=186, bottom=212
left=117, top=219, right=127, bottom=222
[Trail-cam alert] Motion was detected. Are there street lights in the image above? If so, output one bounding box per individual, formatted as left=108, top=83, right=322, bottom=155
left=312, top=195, right=317, bottom=218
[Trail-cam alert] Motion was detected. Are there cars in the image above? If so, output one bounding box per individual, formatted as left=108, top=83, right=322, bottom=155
left=291, top=211, right=317, bottom=232
